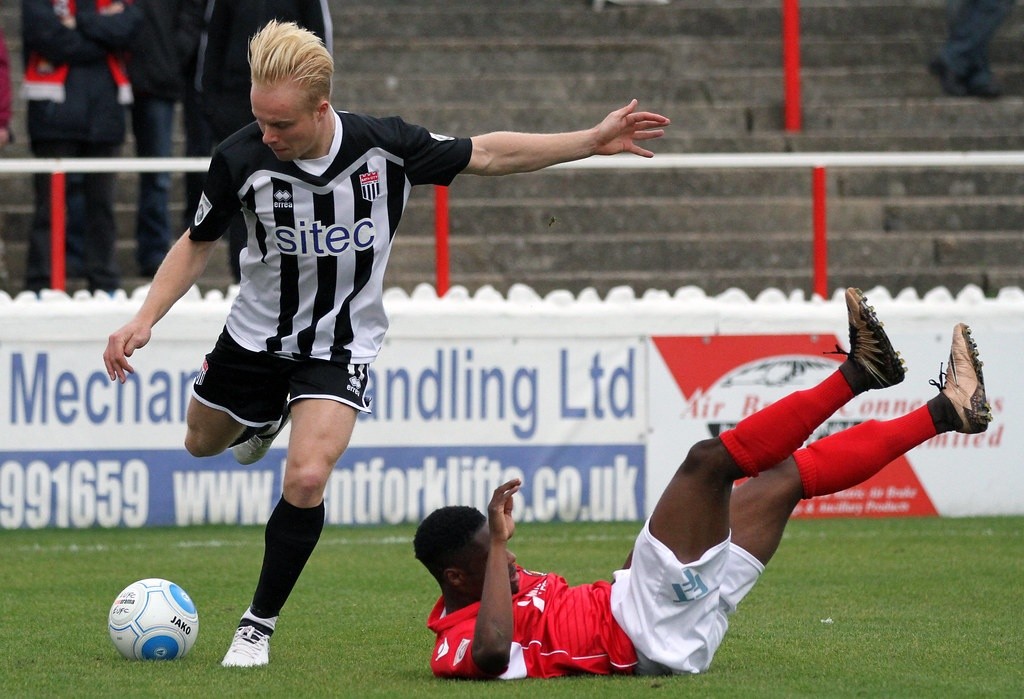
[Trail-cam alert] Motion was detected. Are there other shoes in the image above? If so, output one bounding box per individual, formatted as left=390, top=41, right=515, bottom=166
left=140, top=262, right=160, bottom=279
left=926, top=58, right=1003, bottom=99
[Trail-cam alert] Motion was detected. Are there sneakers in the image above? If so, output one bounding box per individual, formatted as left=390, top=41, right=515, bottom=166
left=927, top=323, right=990, bottom=434
left=219, top=625, right=270, bottom=669
left=822, top=288, right=907, bottom=387
left=232, top=404, right=292, bottom=464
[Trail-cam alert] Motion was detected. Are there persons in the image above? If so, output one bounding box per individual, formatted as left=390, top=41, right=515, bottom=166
left=412, top=287, right=991, bottom=680
left=196, top=0, right=334, bottom=282
left=928, top=1, right=1016, bottom=100
left=103, top=20, right=668, bottom=666
left=0, top=0, right=208, bottom=297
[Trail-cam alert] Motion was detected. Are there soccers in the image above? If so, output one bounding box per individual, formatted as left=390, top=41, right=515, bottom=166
left=107, top=577, right=200, bottom=662
